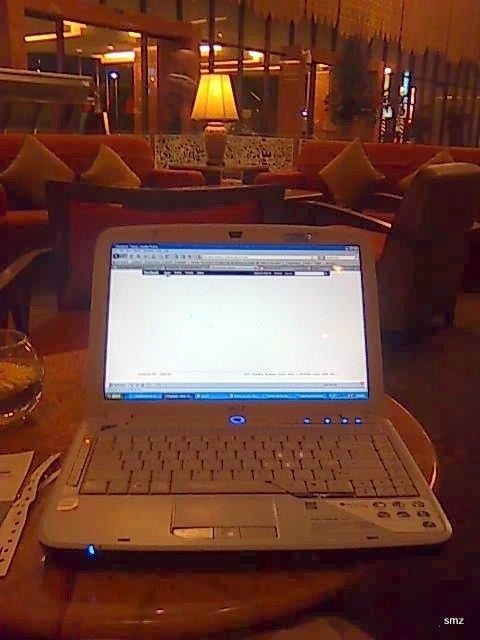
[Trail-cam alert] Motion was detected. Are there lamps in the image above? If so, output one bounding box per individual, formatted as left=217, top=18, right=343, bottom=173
left=191, top=74, right=240, bottom=164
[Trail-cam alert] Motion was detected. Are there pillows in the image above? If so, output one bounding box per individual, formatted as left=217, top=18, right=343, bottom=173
left=398, top=148, right=458, bottom=197
left=318, top=138, right=387, bottom=207
left=5, top=133, right=78, bottom=211
left=81, top=144, right=141, bottom=188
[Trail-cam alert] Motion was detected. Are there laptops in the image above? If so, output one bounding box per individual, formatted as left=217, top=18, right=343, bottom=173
left=38, top=222, right=453, bottom=553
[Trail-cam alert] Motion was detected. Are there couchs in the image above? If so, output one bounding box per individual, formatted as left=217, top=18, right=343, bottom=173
left=0, top=131, right=205, bottom=275
left=255, top=136, right=479, bottom=295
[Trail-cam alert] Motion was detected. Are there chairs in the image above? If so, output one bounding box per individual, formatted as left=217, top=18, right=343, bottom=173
left=330, top=163, right=480, bottom=342
left=0, top=180, right=283, bottom=353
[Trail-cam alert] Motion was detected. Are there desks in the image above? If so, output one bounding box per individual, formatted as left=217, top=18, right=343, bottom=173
left=0, top=303, right=480, bottom=640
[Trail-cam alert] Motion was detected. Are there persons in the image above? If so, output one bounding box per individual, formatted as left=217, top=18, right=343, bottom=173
left=77, top=94, right=101, bottom=134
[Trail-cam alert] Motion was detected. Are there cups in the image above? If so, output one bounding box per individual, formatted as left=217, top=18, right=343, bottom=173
left=0, top=328, right=46, bottom=428
